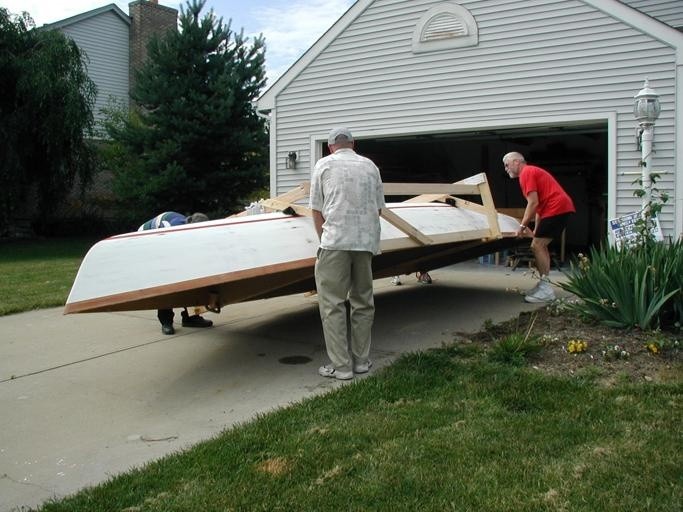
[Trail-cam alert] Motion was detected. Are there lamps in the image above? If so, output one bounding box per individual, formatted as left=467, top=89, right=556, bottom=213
left=286, top=151, right=297, bottom=169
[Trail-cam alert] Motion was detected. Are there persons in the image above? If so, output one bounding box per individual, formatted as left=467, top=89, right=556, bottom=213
left=304, top=125, right=387, bottom=381
left=134, top=209, right=216, bottom=336
left=500, top=149, right=577, bottom=305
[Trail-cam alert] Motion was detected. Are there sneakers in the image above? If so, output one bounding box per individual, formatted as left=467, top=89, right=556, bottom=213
left=354, top=359, right=372, bottom=373
left=391, top=276, right=402, bottom=286
left=182, top=315, right=213, bottom=328
left=161, top=323, right=175, bottom=334
left=521, top=281, right=555, bottom=303
left=319, top=365, right=353, bottom=380
left=417, top=274, right=432, bottom=283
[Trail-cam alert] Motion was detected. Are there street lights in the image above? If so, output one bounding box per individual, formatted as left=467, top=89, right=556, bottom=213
left=632, top=74, right=661, bottom=254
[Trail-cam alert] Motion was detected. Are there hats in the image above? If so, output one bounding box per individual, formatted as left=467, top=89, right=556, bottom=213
left=327, top=126, right=353, bottom=144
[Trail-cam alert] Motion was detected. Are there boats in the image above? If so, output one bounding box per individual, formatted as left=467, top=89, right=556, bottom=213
left=63, top=203, right=538, bottom=312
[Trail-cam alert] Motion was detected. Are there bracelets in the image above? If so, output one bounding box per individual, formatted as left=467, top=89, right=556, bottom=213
left=518, top=224, right=527, bottom=229
